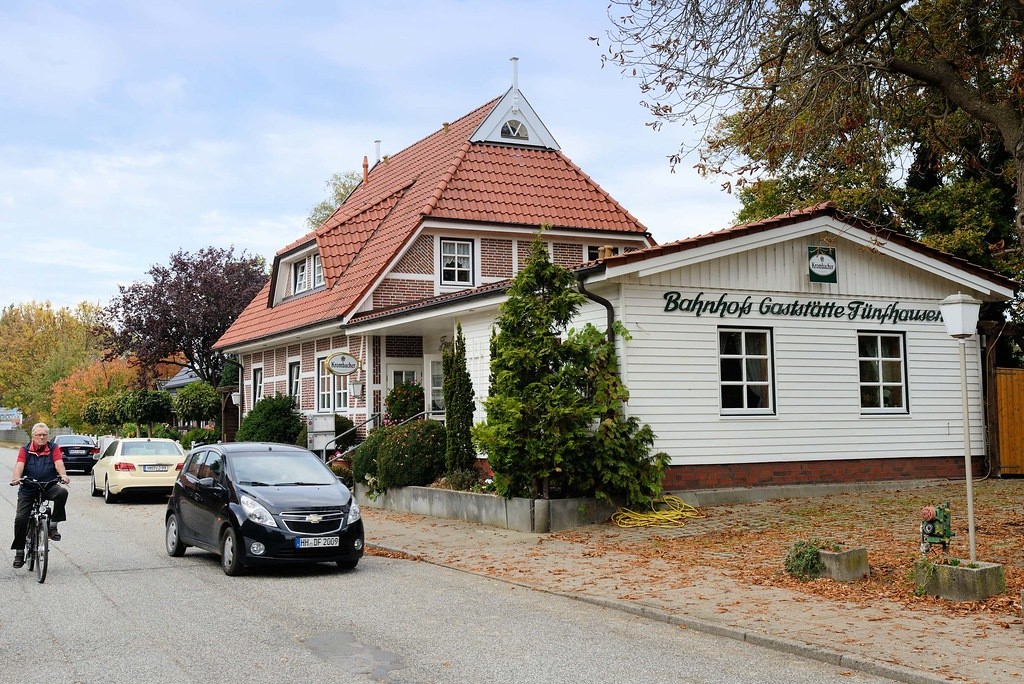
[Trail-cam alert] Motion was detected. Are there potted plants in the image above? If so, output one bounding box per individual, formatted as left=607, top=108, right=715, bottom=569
left=905, top=553, right=1003, bottom=603
left=782, top=538, right=871, bottom=584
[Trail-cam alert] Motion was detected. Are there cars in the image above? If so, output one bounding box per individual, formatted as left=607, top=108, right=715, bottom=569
left=49, top=435, right=100, bottom=475
left=165, top=443, right=365, bottom=575
left=90, top=437, right=186, bottom=505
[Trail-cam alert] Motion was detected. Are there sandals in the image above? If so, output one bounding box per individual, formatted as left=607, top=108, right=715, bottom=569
left=49, top=526, right=61, bottom=540
left=13, top=552, right=24, bottom=568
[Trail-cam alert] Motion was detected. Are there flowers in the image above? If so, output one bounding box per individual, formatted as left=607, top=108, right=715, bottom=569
left=329, top=451, right=351, bottom=461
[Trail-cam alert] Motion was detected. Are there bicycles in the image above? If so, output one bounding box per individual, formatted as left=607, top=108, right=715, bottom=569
left=10, top=475, right=71, bottom=584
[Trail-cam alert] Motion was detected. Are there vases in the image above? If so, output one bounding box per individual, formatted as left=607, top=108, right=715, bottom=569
left=331, top=459, right=350, bottom=471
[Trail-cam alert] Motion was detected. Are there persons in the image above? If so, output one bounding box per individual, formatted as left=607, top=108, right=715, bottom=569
left=10, top=422, right=70, bottom=569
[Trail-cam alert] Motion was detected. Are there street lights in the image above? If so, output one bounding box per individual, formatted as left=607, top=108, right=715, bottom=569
left=936, top=289, right=983, bottom=563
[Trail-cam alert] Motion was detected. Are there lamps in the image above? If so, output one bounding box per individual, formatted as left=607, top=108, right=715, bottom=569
left=348, top=379, right=364, bottom=403
left=230, top=387, right=240, bottom=406
